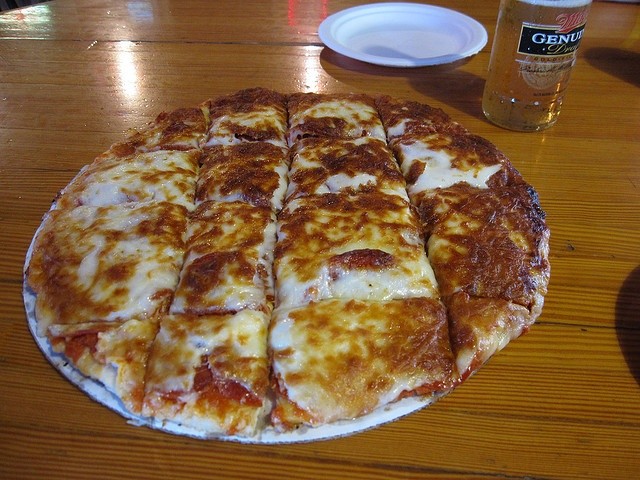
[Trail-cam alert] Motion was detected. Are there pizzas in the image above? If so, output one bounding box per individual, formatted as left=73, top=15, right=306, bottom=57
left=26, top=87, right=552, bottom=438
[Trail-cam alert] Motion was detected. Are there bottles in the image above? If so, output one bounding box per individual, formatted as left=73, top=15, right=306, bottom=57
left=480, top=0, right=593, bottom=132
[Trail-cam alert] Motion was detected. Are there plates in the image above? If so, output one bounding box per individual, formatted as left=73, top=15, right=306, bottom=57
left=317, top=1, right=488, bottom=68
